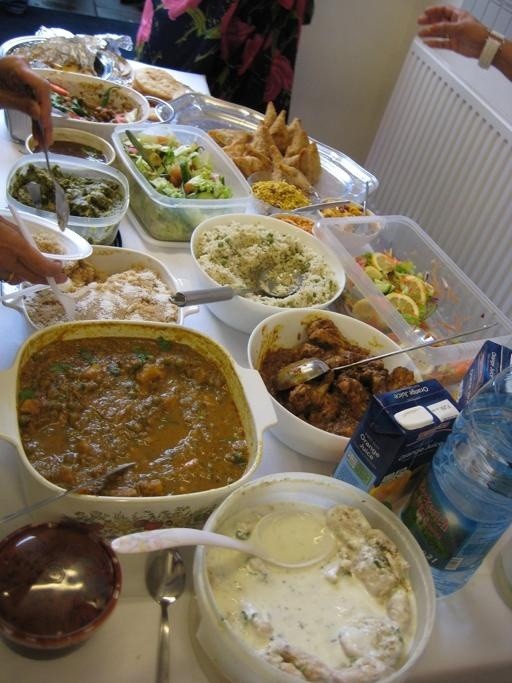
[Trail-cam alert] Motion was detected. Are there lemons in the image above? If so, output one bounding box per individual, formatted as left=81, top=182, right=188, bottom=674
left=352, top=252, right=425, bottom=327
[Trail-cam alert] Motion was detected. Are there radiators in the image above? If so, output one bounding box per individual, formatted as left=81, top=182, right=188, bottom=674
left=357, top=36, right=512, bottom=331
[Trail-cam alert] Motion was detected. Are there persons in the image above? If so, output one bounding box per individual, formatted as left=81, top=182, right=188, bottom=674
left=1, top=51, right=75, bottom=288
left=136, top=0, right=313, bottom=124
left=415, top=4, right=511, bottom=83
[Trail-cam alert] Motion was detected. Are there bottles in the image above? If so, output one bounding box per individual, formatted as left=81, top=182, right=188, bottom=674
left=397, top=366, right=512, bottom=596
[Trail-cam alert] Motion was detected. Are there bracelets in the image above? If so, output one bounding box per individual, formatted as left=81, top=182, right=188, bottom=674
left=475, top=29, right=506, bottom=69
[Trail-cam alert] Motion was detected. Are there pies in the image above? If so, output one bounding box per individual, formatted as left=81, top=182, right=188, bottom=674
left=131, top=68, right=183, bottom=101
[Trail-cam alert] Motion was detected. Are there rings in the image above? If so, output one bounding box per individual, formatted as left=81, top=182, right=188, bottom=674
left=5, top=270, right=17, bottom=283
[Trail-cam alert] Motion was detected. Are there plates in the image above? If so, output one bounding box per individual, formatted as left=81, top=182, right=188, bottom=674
left=164, top=91, right=379, bottom=211
left=0, top=32, right=113, bottom=78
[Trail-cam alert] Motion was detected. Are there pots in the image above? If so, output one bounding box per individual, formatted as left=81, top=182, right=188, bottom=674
left=0, top=317, right=281, bottom=529
left=2, top=242, right=198, bottom=334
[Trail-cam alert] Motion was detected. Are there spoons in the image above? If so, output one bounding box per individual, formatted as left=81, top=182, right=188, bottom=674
left=32, top=113, right=73, bottom=234
left=143, top=545, right=189, bottom=682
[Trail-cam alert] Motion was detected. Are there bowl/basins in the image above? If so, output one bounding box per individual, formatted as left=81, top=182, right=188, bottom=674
left=190, top=469, right=434, bottom=683
left=190, top=212, right=346, bottom=328
left=22, top=124, right=115, bottom=167
left=5, top=152, right=131, bottom=246
left=314, top=215, right=511, bottom=383
left=9, top=65, right=150, bottom=146
left=110, top=121, right=254, bottom=240
left=140, top=91, right=173, bottom=125
left=1, top=519, right=120, bottom=658
left=243, top=310, right=422, bottom=467
left=243, top=165, right=318, bottom=208
